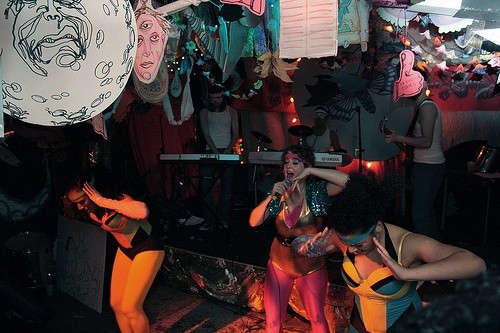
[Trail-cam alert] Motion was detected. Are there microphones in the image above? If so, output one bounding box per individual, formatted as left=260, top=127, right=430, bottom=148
left=266, top=178, right=292, bottom=208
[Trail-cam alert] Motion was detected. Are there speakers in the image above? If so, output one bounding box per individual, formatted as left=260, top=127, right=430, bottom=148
left=52, top=214, right=118, bottom=314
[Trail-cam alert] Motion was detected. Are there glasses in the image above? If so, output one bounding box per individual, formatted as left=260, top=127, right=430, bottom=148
left=312, top=113, right=327, bottom=119
left=208, top=95, right=223, bottom=101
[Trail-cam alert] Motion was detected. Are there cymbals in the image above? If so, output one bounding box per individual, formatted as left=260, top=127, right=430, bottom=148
left=288, top=125, right=314, bottom=138
left=250, top=130, right=272, bottom=144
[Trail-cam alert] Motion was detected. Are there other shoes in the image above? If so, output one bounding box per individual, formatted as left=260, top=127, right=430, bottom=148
left=199, top=222, right=216, bottom=232
left=219, top=223, right=229, bottom=230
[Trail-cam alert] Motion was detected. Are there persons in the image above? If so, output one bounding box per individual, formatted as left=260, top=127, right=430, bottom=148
left=288, top=171, right=486, bottom=332
left=197, top=87, right=239, bottom=229
left=384, top=65, right=445, bottom=237
left=304, top=107, right=340, bottom=153
left=59, top=168, right=165, bottom=333
left=248, top=144, right=356, bottom=333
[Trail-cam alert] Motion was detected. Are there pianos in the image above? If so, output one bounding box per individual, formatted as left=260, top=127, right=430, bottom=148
left=157, top=152, right=241, bottom=228
left=247, top=151, right=354, bottom=167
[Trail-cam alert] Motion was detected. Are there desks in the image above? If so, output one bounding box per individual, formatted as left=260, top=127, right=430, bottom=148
left=440, top=171, right=500, bottom=243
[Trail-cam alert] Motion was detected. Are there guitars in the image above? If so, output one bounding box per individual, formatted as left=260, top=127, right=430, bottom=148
left=379, top=116, right=407, bottom=153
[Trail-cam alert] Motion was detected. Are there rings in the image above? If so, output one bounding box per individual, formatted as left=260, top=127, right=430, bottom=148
left=276, top=186, right=279, bottom=190
